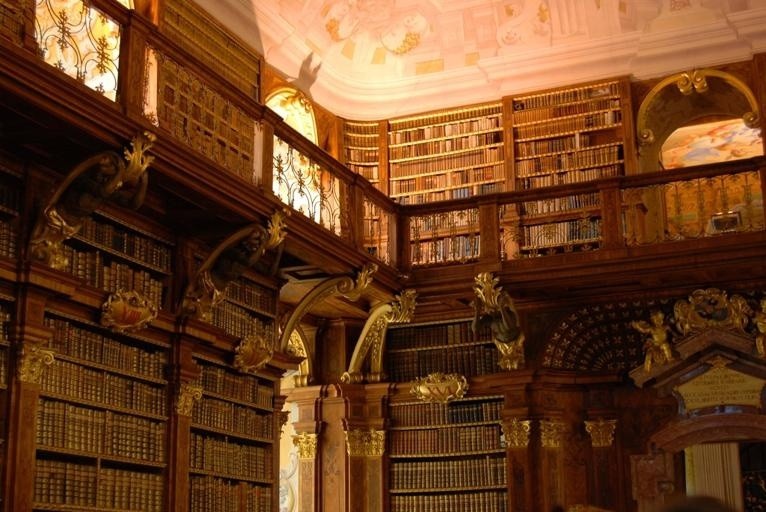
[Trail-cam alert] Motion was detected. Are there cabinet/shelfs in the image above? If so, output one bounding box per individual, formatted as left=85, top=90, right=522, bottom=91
left=0, top=160, right=31, bottom=510
left=388, top=95, right=508, bottom=271
left=379, top=318, right=511, bottom=512
left=153, top=1, right=261, bottom=185
left=338, top=114, right=388, bottom=264
left=508, top=75, right=632, bottom=263
left=179, top=236, right=278, bottom=512
left=32, top=171, right=177, bottom=511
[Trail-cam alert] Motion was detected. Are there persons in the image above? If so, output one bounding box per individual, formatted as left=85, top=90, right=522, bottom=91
left=631, top=313, right=676, bottom=370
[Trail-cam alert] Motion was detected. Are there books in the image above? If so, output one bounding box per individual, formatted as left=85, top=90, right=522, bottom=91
left=680, top=440, right=746, bottom=512
left=518, top=192, right=601, bottom=247
left=191, top=358, right=274, bottom=409
left=209, top=278, right=276, bottom=340
left=33, top=460, right=163, bottom=511
left=191, top=397, right=273, bottom=441
left=343, top=122, right=379, bottom=190
left=40, top=318, right=168, bottom=417
left=190, top=476, right=272, bottom=511
left=37, top=399, right=164, bottom=463
left=511, top=82, right=621, bottom=189
left=190, top=433, right=272, bottom=480
left=362, top=196, right=380, bottom=256
left=0, top=312, right=12, bottom=391
left=389, top=106, right=505, bottom=206
left=0, top=213, right=173, bottom=311
left=409, top=205, right=505, bottom=264
left=382, top=317, right=506, bottom=384
left=390, top=400, right=504, bottom=454
left=390, top=457, right=509, bottom=511
